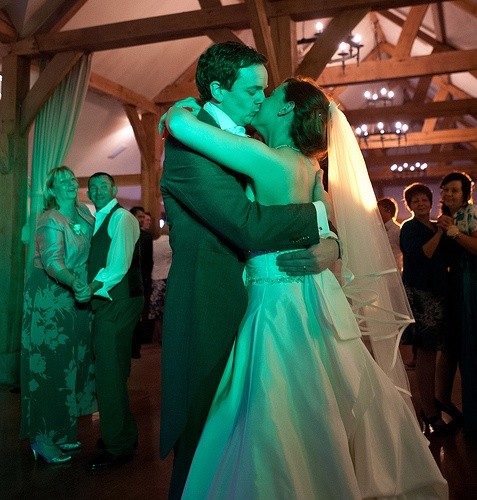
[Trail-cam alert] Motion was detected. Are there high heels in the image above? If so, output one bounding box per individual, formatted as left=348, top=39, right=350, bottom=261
left=57, top=441, right=82, bottom=451
left=31, top=441, right=72, bottom=465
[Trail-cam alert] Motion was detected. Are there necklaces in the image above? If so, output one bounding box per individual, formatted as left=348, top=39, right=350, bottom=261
left=276, top=145, right=301, bottom=153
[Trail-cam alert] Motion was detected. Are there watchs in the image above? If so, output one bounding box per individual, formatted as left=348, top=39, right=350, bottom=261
left=329, top=236, right=344, bottom=259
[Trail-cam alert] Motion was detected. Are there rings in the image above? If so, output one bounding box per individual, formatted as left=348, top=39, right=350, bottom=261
left=304, top=266, right=306, bottom=271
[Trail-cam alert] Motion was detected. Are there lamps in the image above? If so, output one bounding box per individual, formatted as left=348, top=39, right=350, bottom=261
left=295, top=19, right=363, bottom=68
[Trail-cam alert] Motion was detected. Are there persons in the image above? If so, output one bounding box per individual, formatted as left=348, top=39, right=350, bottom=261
left=158, top=43, right=345, bottom=500
left=19, top=166, right=477, bottom=466
left=161, top=76, right=454, bottom=500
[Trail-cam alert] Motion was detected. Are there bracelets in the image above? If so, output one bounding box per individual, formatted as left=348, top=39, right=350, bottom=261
left=447, top=223, right=458, bottom=236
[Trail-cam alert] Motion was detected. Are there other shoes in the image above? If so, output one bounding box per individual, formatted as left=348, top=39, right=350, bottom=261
left=97, top=438, right=108, bottom=449
left=404, top=362, right=416, bottom=369
left=85, top=457, right=128, bottom=476
